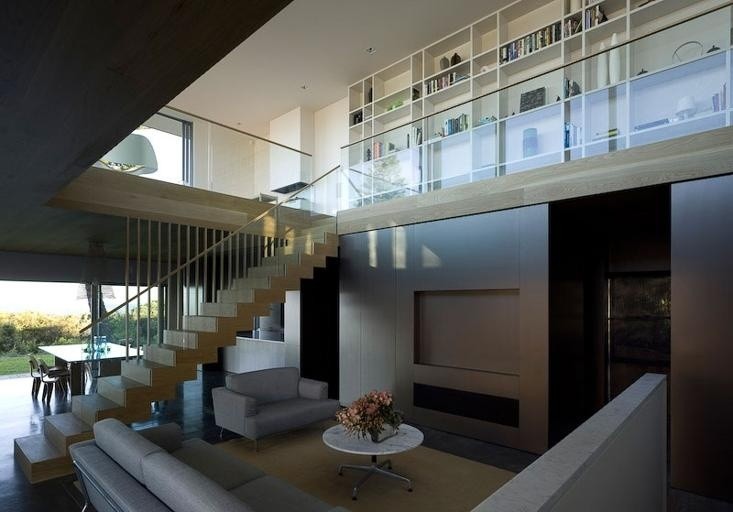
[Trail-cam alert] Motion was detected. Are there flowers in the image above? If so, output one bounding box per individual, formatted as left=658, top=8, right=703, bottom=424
left=333, top=390, right=403, bottom=441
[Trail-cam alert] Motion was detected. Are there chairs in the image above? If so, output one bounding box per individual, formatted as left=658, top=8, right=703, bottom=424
left=27, top=353, right=70, bottom=402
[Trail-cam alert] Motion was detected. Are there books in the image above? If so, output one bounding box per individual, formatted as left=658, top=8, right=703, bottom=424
left=424, top=72, right=458, bottom=97
left=565, top=120, right=619, bottom=149
left=711, top=82, right=726, bottom=115
left=499, top=1, right=609, bottom=65
left=373, top=125, right=422, bottom=160
left=442, top=112, right=467, bottom=138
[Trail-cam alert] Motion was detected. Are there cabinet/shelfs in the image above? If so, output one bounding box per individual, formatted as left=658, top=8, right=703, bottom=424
left=347, top=1, right=732, bottom=209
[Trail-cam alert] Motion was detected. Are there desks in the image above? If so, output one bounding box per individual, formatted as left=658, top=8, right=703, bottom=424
left=36, top=342, right=144, bottom=397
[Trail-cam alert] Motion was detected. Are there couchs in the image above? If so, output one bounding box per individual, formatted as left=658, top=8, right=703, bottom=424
left=211, top=367, right=339, bottom=452
left=68, top=417, right=350, bottom=512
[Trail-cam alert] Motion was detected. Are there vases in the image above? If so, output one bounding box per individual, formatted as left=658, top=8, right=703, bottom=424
left=367, top=429, right=378, bottom=442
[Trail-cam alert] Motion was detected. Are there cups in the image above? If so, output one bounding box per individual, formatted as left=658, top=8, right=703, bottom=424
left=93, top=335, right=107, bottom=352
